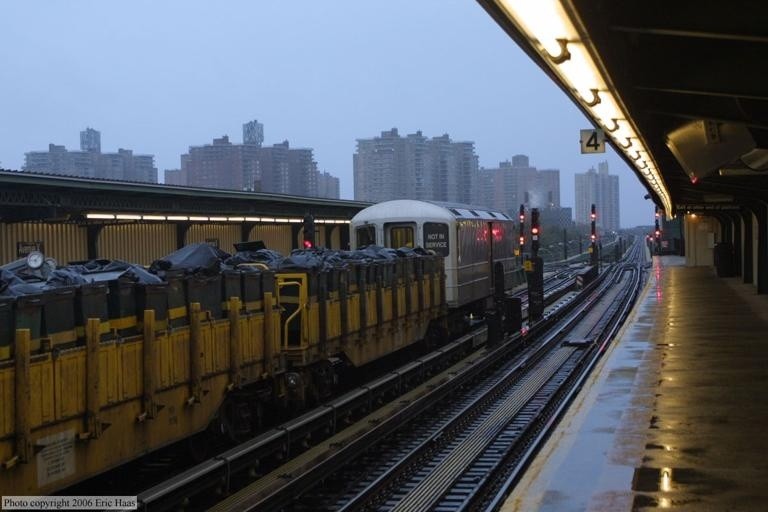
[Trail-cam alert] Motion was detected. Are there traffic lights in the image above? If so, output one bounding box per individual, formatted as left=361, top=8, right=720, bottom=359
left=302, top=215, right=317, bottom=250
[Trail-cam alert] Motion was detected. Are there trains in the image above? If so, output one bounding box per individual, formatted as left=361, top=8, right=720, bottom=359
left=0, top=197, right=524, bottom=510
